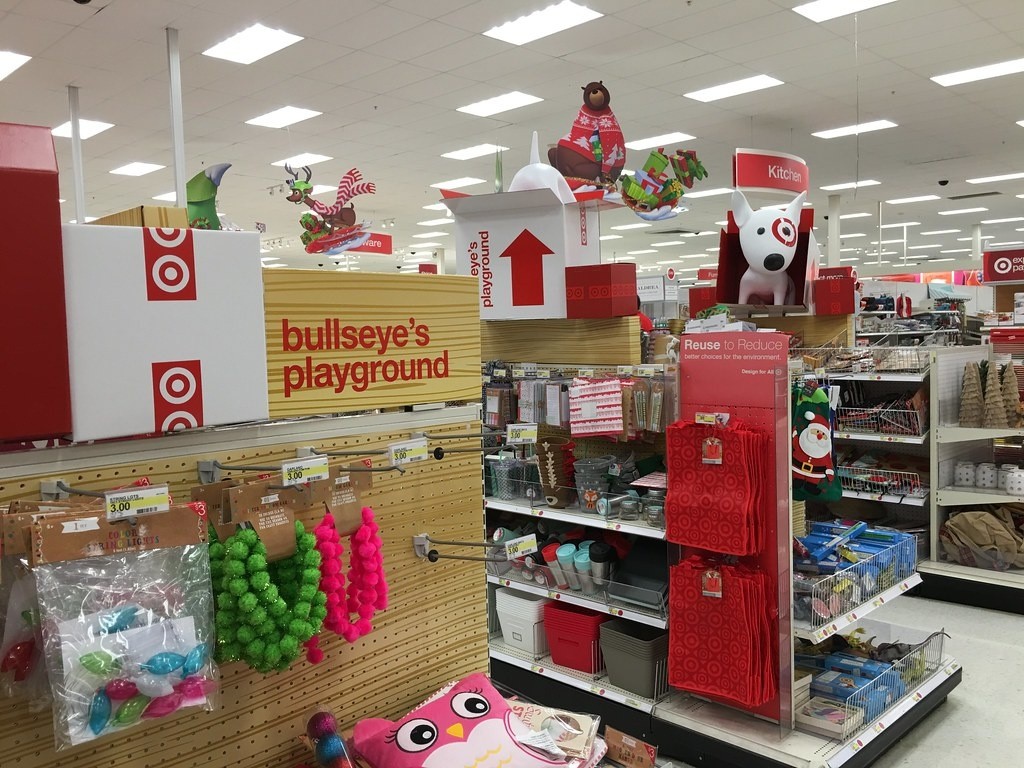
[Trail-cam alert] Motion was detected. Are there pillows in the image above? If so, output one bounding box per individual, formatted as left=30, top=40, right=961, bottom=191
left=354, top=674, right=567, bottom=768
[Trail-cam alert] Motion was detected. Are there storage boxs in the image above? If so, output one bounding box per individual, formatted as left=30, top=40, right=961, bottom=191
left=813, top=278, right=855, bottom=317
left=84, top=205, right=189, bottom=228
left=794, top=651, right=906, bottom=741
left=494, top=587, right=668, bottom=699
left=60, top=221, right=269, bottom=444
left=0, top=122, right=73, bottom=442
left=714, top=207, right=821, bottom=315
left=566, top=262, right=638, bottom=319
left=689, top=287, right=716, bottom=319
left=983, top=292, right=1024, bottom=326
left=794, top=518, right=916, bottom=578
left=436, top=187, right=625, bottom=322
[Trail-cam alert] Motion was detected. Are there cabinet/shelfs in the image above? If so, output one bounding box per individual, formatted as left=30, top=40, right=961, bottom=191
left=804, top=364, right=930, bottom=598
left=488, top=360, right=962, bottom=768
left=930, top=343, right=1024, bottom=615
